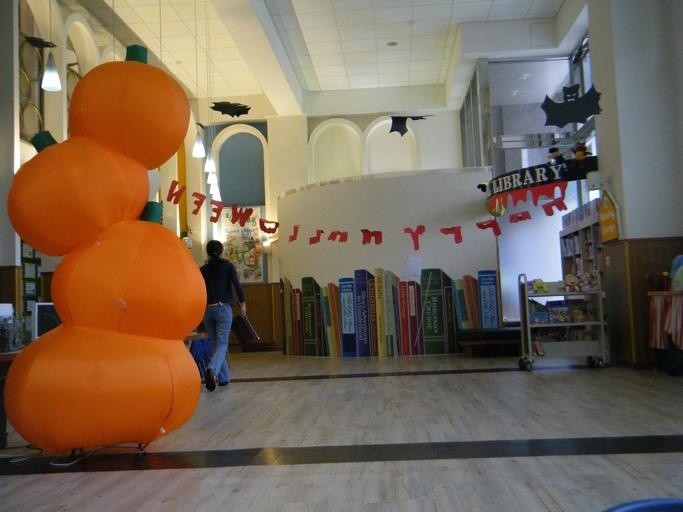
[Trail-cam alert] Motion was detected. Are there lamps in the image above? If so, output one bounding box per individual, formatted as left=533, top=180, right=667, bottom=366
left=39, top=1, right=61, bottom=95
left=189, top=0, right=224, bottom=203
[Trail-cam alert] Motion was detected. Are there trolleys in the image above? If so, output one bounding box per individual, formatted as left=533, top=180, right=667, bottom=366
left=518, top=270, right=609, bottom=371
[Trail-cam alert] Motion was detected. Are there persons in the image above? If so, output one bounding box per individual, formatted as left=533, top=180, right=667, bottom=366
left=199, top=240, right=246, bottom=392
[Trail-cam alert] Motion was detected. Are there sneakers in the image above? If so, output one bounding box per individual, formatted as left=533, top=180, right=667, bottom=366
left=206, top=369, right=216, bottom=391
left=217, top=381, right=228, bottom=386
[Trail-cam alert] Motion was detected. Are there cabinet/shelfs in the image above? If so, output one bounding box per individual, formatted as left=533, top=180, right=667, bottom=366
left=517, top=271, right=611, bottom=358
left=555, top=216, right=605, bottom=281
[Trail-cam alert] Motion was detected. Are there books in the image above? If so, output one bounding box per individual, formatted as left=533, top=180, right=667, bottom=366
left=283, top=266, right=498, bottom=357
left=560, top=197, right=601, bottom=271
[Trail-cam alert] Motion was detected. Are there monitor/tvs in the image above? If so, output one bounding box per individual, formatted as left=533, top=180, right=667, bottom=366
left=32, top=303, right=62, bottom=341
